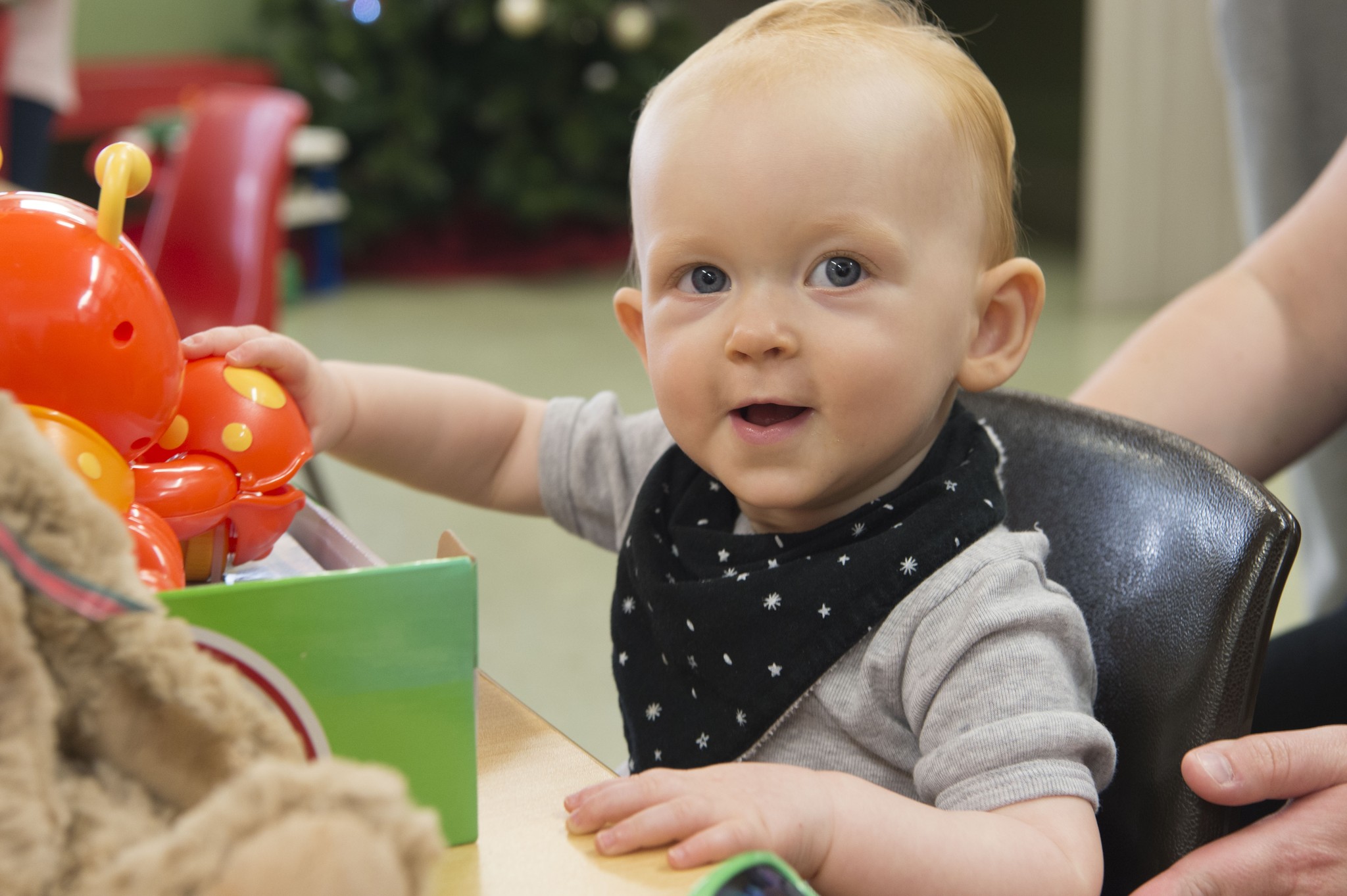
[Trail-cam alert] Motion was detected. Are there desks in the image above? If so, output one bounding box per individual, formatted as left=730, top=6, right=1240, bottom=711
left=225, top=533, right=719, bottom=896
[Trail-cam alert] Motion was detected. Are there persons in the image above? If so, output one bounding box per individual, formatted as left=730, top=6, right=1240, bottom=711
left=1068, top=140, right=1347, bottom=895
left=184, top=0, right=1118, bottom=896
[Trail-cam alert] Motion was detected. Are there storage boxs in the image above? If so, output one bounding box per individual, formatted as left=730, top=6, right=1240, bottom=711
left=153, top=489, right=481, bottom=858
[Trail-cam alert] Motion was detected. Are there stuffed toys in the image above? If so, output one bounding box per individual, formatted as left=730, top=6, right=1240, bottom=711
left=1, top=392, right=442, bottom=895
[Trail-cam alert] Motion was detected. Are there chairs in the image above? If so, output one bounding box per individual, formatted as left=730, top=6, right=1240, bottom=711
left=954, top=383, right=1302, bottom=894
left=132, top=78, right=345, bottom=527
